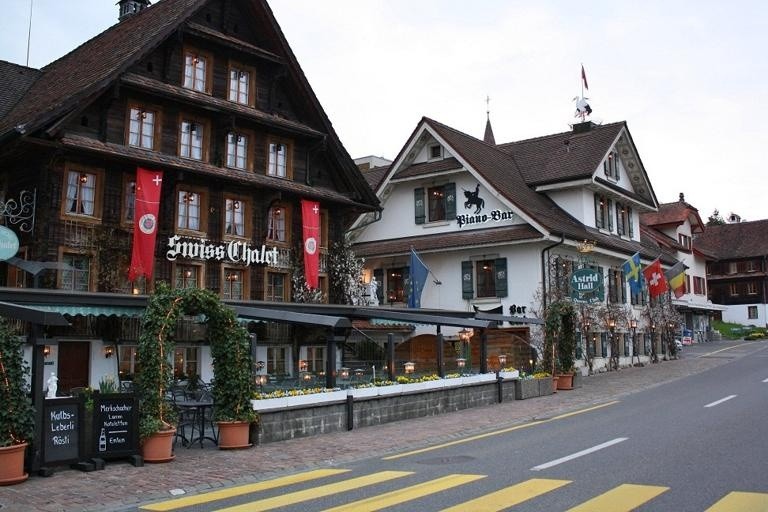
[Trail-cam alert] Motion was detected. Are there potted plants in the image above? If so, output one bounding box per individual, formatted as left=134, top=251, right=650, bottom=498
left=0, top=312, right=31, bottom=485
left=544, top=297, right=578, bottom=392
left=138, top=286, right=257, bottom=461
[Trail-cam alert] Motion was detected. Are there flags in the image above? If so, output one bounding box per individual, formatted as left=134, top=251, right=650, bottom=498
left=127, top=164, right=167, bottom=283
left=405, top=251, right=431, bottom=308
left=581, top=65, right=589, bottom=90
left=623, top=253, right=648, bottom=296
left=663, top=262, right=687, bottom=299
left=640, top=260, right=669, bottom=299
left=299, top=199, right=322, bottom=292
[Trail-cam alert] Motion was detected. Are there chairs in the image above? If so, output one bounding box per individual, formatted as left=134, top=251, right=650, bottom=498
left=124, top=366, right=374, bottom=449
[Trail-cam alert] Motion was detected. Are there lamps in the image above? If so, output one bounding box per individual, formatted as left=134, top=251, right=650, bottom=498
left=106, top=344, right=115, bottom=355
left=578, top=239, right=597, bottom=253
left=433, top=191, right=443, bottom=200
left=234, top=201, right=239, bottom=208
left=80, top=171, right=87, bottom=182
left=277, top=143, right=281, bottom=151
left=240, top=71, right=243, bottom=78
left=456, top=357, right=466, bottom=367
left=275, top=207, right=281, bottom=214
left=237, top=133, right=241, bottom=142
left=196, top=56, right=199, bottom=63
left=458, top=328, right=470, bottom=342
left=498, top=355, right=507, bottom=364
left=184, top=267, right=192, bottom=278
left=44, top=347, right=49, bottom=355
left=189, top=193, right=194, bottom=201
left=231, top=271, right=239, bottom=280
left=404, top=361, right=415, bottom=373
left=191, top=123, right=198, bottom=131
left=483, top=263, right=489, bottom=270
left=142, top=110, right=147, bottom=117
left=610, top=316, right=616, bottom=328
left=631, top=316, right=639, bottom=328
